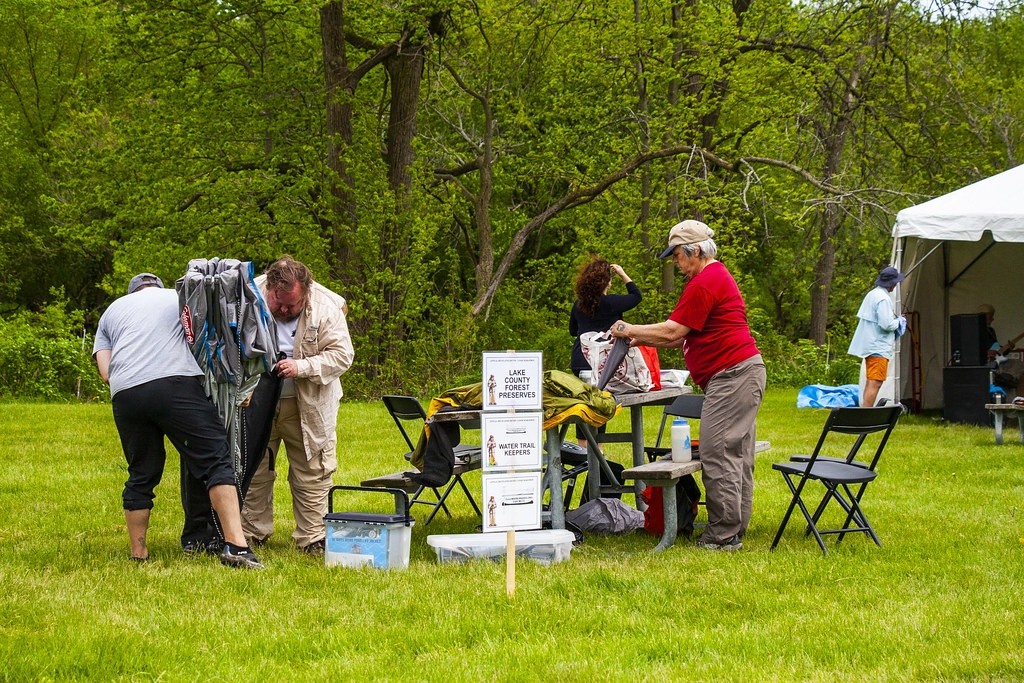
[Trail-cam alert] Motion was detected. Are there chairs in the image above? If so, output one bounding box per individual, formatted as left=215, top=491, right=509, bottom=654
left=382, top=395, right=482, bottom=526
left=644, top=395, right=706, bottom=463
left=770, top=398, right=903, bottom=557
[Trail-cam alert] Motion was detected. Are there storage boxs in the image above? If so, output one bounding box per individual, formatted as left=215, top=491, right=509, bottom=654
left=323, top=486, right=417, bottom=572
left=427, top=529, right=577, bottom=564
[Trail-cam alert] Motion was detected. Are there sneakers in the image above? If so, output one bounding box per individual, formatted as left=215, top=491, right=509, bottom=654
left=220, top=544, right=265, bottom=570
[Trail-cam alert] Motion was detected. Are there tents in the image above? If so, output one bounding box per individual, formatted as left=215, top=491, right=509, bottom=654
left=858, top=163, right=1024, bottom=410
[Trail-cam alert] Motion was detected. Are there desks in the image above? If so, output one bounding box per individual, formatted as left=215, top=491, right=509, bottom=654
left=431, top=386, right=693, bottom=530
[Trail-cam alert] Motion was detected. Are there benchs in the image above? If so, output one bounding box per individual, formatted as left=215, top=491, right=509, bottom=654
left=361, top=459, right=482, bottom=515
left=986, top=404, right=1024, bottom=445
left=622, top=441, right=770, bottom=553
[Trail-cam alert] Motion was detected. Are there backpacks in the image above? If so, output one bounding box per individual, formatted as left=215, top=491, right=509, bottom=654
left=541, top=370, right=617, bottom=424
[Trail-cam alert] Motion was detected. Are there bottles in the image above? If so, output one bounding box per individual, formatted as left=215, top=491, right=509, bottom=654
left=671, top=416, right=691, bottom=463
left=954, top=351, right=961, bottom=366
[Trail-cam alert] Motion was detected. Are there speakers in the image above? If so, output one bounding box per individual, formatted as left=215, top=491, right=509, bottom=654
left=949, top=313, right=988, bottom=366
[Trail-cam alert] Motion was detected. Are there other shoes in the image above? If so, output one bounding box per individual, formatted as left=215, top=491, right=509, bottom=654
left=305, top=541, right=324, bottom=555
left=698, top=536, right=743, bottom=550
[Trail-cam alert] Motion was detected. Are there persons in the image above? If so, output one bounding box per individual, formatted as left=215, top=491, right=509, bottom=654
left=240, top=254, right=354, bottom=561
left=610, top=221, right=766, bottom=552
left=846, top=267, right=906, bottom=408
left=569, top=257, right=641, bottom=457
left=92, top=273, right=265, bottom=570
left=975, top=303, right=1016, bottom=390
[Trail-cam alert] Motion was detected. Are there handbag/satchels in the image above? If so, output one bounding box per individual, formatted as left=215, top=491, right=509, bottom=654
left=580, top=331, right=655, bottom=395
left=636, top=344, right=663, bottom=391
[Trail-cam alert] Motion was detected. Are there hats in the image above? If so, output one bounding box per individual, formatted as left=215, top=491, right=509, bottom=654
left=128, top=273, right=164, bottom=293
left=659, top=220, right=709, bottom=259
left=874, top=267, right=905, bottom=287
left=976, top=304, right=995, bottom=313
